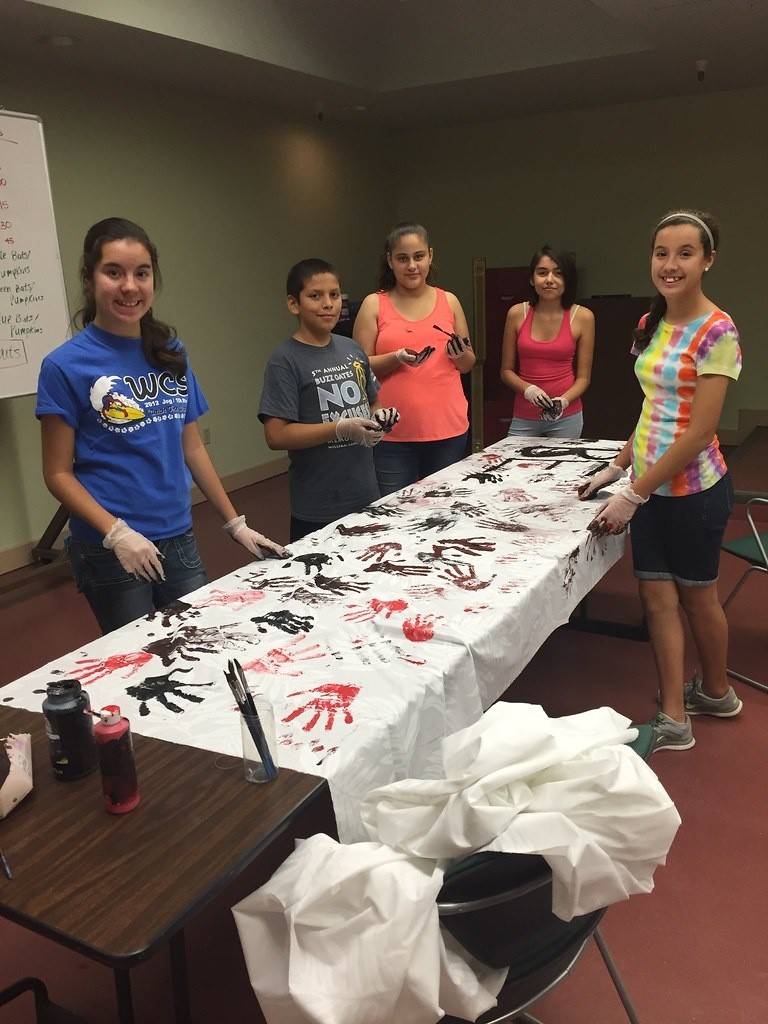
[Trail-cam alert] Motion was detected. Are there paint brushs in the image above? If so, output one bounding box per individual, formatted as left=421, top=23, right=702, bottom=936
left=223, top=658, right=277, bottom=779
left=433, top=325, right=471, bottom=347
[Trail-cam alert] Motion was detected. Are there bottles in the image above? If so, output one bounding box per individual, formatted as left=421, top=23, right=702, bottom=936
left=95, top=705, right=140, bottom=813
left=240, top=701, right=278, bottom=783
left=42, top=680, right=100, bottom=781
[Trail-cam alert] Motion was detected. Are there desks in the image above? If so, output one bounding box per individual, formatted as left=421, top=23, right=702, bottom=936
left=2, top=435, right=631, bottom=1024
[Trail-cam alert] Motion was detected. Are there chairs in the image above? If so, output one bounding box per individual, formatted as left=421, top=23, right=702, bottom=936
left=719, top=491, right=768, bottom=693
left=231, top=701, right=682, bottom=1024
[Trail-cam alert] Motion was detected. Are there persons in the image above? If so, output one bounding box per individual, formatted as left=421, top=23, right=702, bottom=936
left=36, top=217, right=292, bottom=636
left=257, top=259, right=400, bottom=544
left=353, top=222, right=476, bottom=498
left=579, top=210, right=743, bottom=752
left=501, top=246, right=595, bottom=439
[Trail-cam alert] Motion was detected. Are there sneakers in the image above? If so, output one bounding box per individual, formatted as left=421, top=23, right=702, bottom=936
left=656, top=669, right=744, bottom=718
left=645, top=710, right=696, bottom=754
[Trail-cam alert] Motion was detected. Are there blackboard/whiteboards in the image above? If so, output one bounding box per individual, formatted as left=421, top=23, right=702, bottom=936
left=0, top=110, right=74, bottom=399
